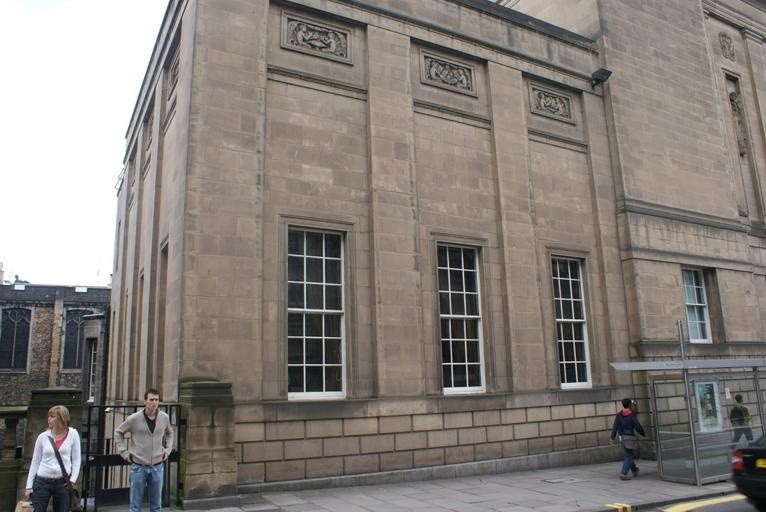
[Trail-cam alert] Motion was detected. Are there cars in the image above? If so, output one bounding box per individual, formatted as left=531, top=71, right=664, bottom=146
left=731, top=431, right=766, bottom=511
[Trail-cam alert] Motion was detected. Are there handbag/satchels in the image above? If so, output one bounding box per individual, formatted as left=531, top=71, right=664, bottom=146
left=621, top=434, right=640, bottom=450
left=71, top=485, right=81, bottom=508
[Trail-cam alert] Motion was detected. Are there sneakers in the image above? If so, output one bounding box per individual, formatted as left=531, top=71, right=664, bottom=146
left=619, top=467, right=639, bottom=480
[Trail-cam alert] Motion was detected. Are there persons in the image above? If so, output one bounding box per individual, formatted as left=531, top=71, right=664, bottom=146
left=728, top=391, right=755, bottom=461
left=23, top=404, right=83, bottom=512
left=110, top=388, right=175, bottom=512
left=607, top=397, right=648, bottom=481
